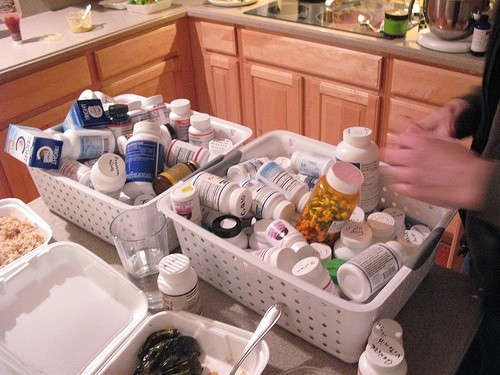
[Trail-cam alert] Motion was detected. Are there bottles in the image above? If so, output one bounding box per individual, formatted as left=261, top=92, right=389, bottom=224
left=367, top=320, right=406, bottom=347
left=36, top=85, right=235, bottom=213
left=357, top=335, right=408, bottom=375
left=383, top=10, right=409, bottom=38
left=168, top=124, right=433, bottom=309
left=0, top=0, right=23, bottom=45
left=469, top=14, right=492, bottom=57
left=156, top=251, right=203, bottom=318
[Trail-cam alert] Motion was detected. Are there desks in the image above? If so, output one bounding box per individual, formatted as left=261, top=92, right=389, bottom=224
left=27, top=195, right=482, bottom=375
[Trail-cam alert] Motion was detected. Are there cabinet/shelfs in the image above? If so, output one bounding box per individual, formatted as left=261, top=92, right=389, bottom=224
left=0, top=16, right=484, bottom=269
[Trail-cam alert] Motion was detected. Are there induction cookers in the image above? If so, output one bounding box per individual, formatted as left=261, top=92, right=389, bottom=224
left=241, top=0, right=423, bottom=40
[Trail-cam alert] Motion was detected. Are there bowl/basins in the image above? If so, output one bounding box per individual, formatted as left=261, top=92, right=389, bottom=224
left=64, top=11, right=93, bottom=32
left=1, top=198, right=271, bottom=374
left=98, top=0, right=172, bottom=13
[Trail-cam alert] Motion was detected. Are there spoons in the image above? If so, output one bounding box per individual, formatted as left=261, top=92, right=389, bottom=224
left=356, top=14, right=379, bottom=34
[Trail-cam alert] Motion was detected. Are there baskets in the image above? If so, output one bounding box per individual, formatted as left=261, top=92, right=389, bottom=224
left=21, top=93, right=461, bottom=364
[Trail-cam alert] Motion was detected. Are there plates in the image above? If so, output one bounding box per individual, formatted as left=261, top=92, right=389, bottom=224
left=207, top=0, right=258, bottom=7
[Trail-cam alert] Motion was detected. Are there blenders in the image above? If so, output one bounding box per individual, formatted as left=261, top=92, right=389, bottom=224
left=415, top=0, right=494, bottom=54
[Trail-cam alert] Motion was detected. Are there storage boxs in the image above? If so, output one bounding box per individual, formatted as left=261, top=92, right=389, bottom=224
left=61, top=98, right=109, bottom=134
left=26, top=92, right=252, bottom=254
left=157, top=128, right=461, bottom=363
left=4, top=123, right=64, bottom=169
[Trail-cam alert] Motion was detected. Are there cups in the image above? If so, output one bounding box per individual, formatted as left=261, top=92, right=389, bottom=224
left=109, top=207, right=176, bottom=311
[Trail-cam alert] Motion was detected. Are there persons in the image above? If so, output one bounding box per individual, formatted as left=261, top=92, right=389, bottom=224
left=381, top=0, right=500, bottom=375
